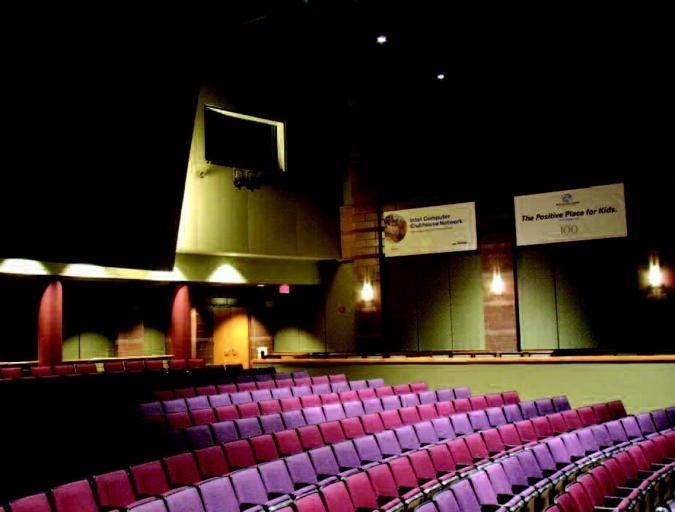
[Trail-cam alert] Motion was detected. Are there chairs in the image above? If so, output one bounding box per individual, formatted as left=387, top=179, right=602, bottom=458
left=268, top=348, right=552, bottom=359
left=0, top=358, right=675, bottom=511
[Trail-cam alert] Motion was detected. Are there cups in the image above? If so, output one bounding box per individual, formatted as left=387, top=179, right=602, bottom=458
left=257, top=347, right=267, bottom=358
